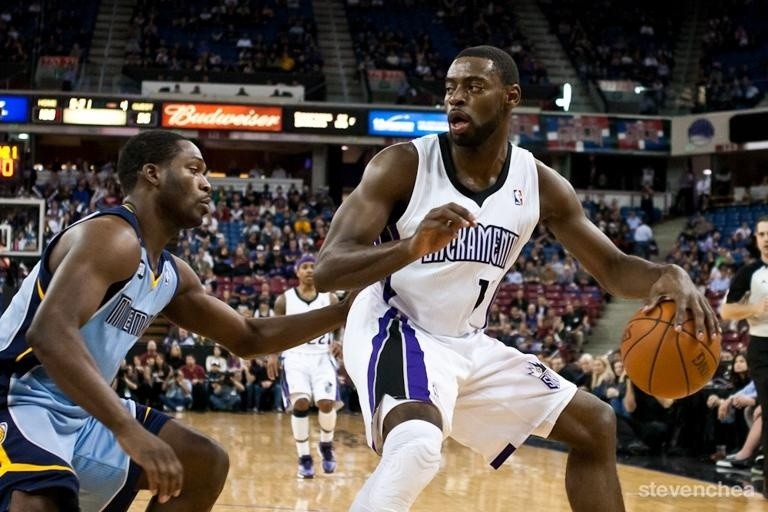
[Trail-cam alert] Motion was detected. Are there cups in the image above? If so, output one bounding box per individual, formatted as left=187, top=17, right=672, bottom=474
left=717, top=445, right=726, bottom=461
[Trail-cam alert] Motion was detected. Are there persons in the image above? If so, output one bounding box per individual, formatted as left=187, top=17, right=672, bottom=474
left=0, top=0, right=768, bottom=512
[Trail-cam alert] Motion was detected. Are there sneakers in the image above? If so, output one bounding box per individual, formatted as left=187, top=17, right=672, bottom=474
left=298, top=455, right=316, bottom=478
left=317, top=442, right=336, bottom=473
left=716, top=455, right=749, bottom=468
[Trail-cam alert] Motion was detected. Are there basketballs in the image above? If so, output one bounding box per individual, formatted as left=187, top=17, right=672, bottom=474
left=620, top=300, right=721, bottom=399
